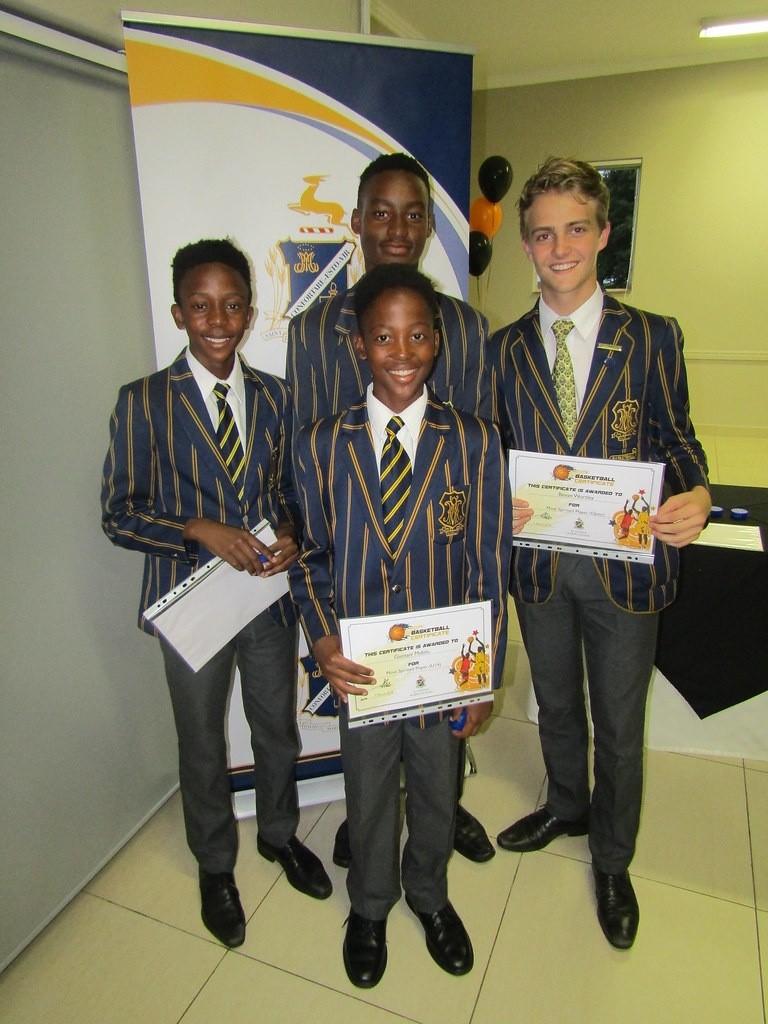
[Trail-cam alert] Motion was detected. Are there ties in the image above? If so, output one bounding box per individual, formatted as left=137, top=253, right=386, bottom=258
left=378, top=415, right=414, bottom=559
left=549, top=319, right=578, bottom=450
left=211, top=382, right=247, bottom=502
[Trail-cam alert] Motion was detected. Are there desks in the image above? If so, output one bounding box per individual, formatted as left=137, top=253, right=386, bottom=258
left=489, top=484, right=768, bottom=760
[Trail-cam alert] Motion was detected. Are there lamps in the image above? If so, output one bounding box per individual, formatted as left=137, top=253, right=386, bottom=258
left=698, top=12, right=768, bottom=38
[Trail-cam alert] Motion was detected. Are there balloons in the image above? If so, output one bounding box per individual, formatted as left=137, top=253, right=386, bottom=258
left=469, top=231, right=492, bottom=276
left=470, top=199, right=503, bottom=241
left=478, top=156, right=512, bottom=206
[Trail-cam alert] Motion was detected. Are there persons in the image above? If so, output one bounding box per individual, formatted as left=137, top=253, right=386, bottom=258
left=288, top=150, right=503, bottom=866
left=286, top=262, right=513, bottom=989
left=100, top=234, right=332, bottom=947
left=489, top=153, right=713, bottom=949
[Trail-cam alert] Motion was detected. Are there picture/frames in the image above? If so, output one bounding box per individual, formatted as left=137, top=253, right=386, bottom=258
left=532, top=158, right=643, bottom=296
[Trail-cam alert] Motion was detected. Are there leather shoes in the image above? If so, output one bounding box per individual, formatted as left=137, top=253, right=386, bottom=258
left=451, top=804, right=496, bottom=863
left=342, top=909, right=389, bottom=989
left=256, top=832, right=333, bottom=900
left=497, top=803, right=595, bottom=851
left=333, top=817, right=354, bottom=868
left=592, top=859, right=640, bottom=948
left=198, top=861, right=247, bottom=947
left=405, top=892, right=475, bottom=977
